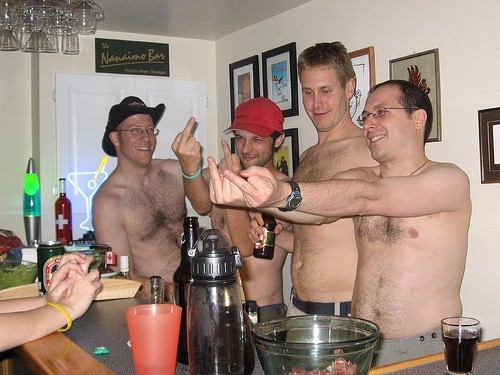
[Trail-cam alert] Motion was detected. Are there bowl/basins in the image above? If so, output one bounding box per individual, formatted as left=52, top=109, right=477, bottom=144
left=251, top=315, right=383, bottom=375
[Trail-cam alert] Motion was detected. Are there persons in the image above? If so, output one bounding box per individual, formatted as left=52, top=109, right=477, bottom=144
left=91, top=96, right=188, bottom=303
left=0, top=252, right=102, bottom=353
left=249, top=41, right=380, bottom=317
left=276, top=156, right=288, bottom=175
left=207, top=80, right=473, bottom=368
left=171, top=96, right=292, bottom=324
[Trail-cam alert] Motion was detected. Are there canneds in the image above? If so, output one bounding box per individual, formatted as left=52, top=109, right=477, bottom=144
left=37, top=240, right=66, bottom=296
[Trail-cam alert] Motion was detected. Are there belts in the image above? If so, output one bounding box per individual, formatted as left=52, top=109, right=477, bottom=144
left=258, top=308, right=283, bottom=322
left=290, top=291, right=352, bottom=317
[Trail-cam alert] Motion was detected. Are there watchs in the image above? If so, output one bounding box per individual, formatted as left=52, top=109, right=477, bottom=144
left=277, top=181, right=302, bottom=212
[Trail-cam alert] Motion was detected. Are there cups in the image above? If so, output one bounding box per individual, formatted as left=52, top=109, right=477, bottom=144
left=124, top=302, right=183, bottom=375
left=1, top=0, right=104, bottom=54
left=140, top=276, right=180, bottom=309
left=441, top=316, right=481, bottom=375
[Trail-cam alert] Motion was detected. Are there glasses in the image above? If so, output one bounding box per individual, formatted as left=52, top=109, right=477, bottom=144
left=357, top=106, right=419, bottom=126
left=112, top=128, right=159, bottom=135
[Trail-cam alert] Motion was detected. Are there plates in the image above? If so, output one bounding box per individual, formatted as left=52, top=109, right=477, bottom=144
left=97, top=270, right=118, bottom=280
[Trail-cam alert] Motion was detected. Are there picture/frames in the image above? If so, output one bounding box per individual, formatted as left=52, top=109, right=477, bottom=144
left=346, top=46, right=376, bottom=129
left=478, top=107, right=500, bottom=183
left=229, top=41, right=300, bottom=187
left=389, top=48, right=442, bottom=142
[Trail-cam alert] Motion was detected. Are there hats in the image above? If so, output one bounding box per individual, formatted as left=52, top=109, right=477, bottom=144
left=101, top=96, right=165, bottom=157
left=223, top=97, right=284, bottom=138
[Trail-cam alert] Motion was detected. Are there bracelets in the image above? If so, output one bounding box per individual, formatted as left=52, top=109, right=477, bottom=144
left=180, top=165, right=201, bottom=179
left=48, top=302, right=72, bottom=332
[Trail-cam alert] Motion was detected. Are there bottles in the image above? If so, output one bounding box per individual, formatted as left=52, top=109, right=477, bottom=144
left=170, top=215, right=204, bottom=365
left=253, top=211, right=275, bottom=260
left=54, top=177, right=74, bottom=245
left=186, top=227, right=248, bottom=374
